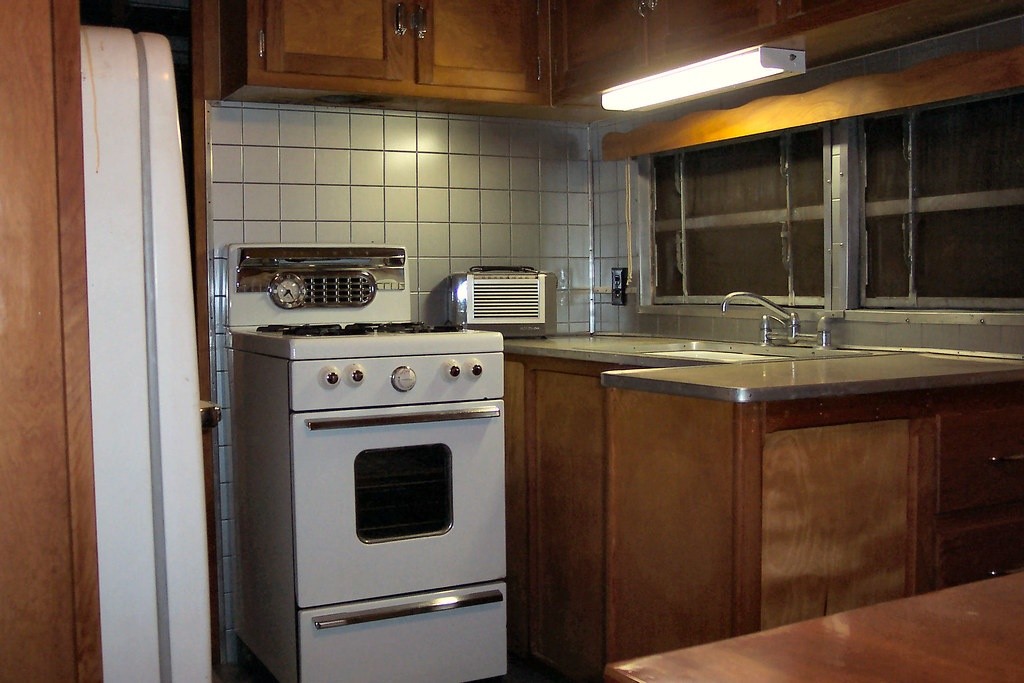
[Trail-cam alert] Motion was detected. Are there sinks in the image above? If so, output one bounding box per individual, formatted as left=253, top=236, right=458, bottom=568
left=622, top=338, right=876, bottom=362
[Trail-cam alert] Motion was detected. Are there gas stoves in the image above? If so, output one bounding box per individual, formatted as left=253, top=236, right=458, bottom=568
left=226, top=242, right=504, bottom=412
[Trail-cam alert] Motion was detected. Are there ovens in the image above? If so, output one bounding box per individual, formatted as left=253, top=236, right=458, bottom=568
left=286, top=400, right=509, bottom=683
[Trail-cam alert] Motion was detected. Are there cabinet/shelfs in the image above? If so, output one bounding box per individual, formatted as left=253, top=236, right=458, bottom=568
left=552, top=0, right=1024, bottom=116
left=222, top=0, right=550, bottom=120
left=504, top=354, right=1024, bottom=683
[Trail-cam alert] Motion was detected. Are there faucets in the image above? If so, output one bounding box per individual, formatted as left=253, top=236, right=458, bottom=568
left=720, top=291, right=838, bottom=349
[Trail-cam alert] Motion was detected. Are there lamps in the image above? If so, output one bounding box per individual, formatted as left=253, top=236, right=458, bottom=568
left=603, top=46, right=806, bottom=113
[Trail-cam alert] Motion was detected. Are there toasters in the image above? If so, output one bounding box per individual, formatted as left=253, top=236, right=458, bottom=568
left=447, top=264, right=559, bottom=341
